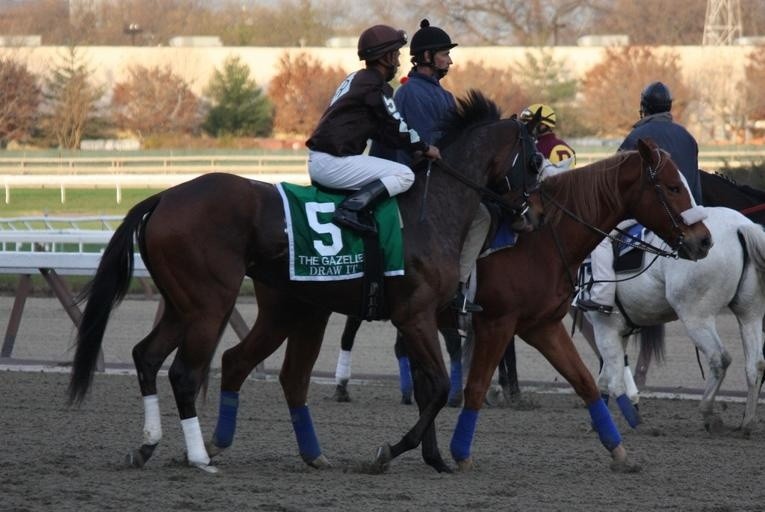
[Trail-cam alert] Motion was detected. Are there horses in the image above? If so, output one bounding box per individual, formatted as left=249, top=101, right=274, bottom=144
left=203, top=137, right=713, bottom=471
left=63, top=89, right=542, bottom=474
left=333, top=155, right=765, bottom=436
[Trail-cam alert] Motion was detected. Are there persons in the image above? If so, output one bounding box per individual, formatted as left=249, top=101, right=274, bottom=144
left=303, top=24, right=442, bottom=236
left=572, top=81, right=702, bottom=315
left=520, top=103, right=575, bottom=170
left=393, top=19, right=458, bottom=149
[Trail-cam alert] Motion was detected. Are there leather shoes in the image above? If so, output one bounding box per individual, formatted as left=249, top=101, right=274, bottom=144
left=578, top=300, right=612, bottom=314
left=450, top=293, right=482, bottom=312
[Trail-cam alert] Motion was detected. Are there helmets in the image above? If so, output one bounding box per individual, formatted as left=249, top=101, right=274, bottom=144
left=410, top=20, right=457, bottom=55
left=520, top=103, right=556, bottom=129
left=358, top=25, right=407, bottom=60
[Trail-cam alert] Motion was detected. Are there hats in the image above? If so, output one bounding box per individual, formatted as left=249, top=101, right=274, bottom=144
left=641, top=82, right=671, bottom=113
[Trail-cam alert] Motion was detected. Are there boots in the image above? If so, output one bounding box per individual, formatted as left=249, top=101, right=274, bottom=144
left=333, top=179, right=390, bottom=236
